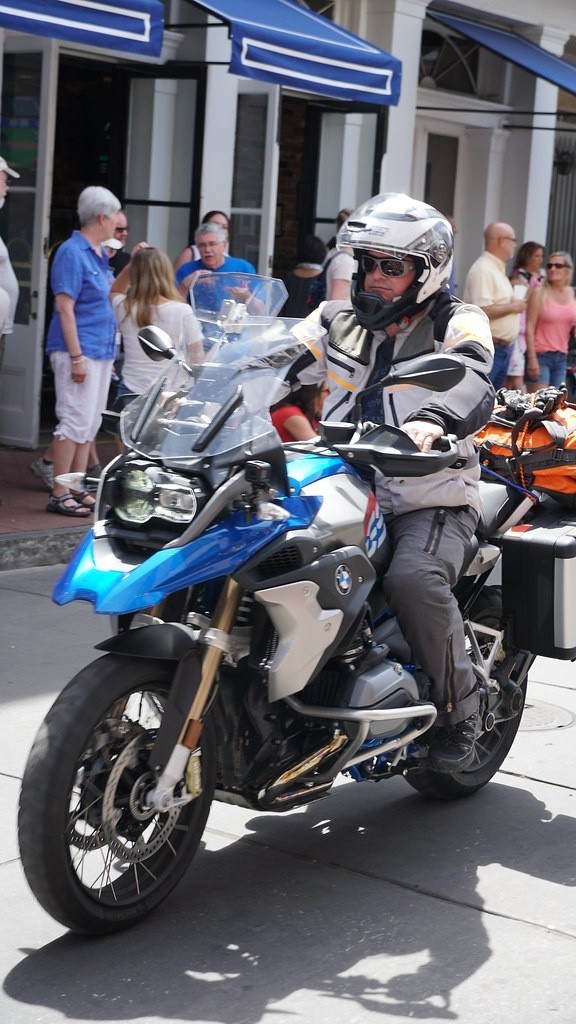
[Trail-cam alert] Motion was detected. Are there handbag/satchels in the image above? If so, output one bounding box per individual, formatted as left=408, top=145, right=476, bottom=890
left=473, top=397, right=576, bottom=509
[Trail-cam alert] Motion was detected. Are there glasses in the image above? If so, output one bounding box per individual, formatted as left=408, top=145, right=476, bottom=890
left=362, top=255, right=416, bottom=278
left=505, top=236, right=517, bottom=242
left=547, top=263, right=570, bottom=269
left=116, top=226, right=130, bottom=233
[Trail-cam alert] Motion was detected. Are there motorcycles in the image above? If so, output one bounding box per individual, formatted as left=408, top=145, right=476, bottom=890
left=15, top=298, right=576, bottom=938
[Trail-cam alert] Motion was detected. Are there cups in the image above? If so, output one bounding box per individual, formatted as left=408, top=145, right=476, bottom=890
left=513, top=284, right=528, bottom=299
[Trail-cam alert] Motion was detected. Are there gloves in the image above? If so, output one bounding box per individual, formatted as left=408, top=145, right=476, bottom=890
left=496, top=381, right=568, bottom=418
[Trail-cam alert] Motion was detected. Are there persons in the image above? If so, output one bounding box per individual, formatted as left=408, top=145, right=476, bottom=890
left=173, top=211, right=265, bottom=351
left=206, top=194, right=495, bottom=768
left=274, top=210, right=357, bottom=448
left=441, top=216, right=576, bottom=401
left=0, top=156, right=21, bottom=375
left=109, top=240, right=202, bottom=406
left=30, top=186, right=132, bottom=516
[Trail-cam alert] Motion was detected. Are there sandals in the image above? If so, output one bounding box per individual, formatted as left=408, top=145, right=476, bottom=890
left=70, top=491, right=95, bottom=511
left=46, top=493, right=92, bottom=516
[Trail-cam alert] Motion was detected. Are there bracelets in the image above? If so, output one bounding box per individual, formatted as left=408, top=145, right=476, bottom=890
left=73, top=358, right=84, bottom=364
left=71, top=354, right=82, bottom=358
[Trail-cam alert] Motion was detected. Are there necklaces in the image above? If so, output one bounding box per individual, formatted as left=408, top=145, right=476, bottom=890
left=396, top=317, right=409, bottom=329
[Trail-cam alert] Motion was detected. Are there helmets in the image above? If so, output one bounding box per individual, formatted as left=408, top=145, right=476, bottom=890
left=336, top=192, right=454, bottom=331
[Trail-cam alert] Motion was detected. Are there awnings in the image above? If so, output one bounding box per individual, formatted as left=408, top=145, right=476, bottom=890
left=416, top=7, right=576, bottom=131
left=0, top=0, right=165, bottom=57
left=164, top=0, right=402, bottom=107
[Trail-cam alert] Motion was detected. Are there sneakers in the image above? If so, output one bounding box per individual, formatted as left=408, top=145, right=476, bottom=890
left=425, top=710, right=481, bottom=772
left=29, top=458, right=55, bottom=489
left=85, top=464, right=103, bottom=492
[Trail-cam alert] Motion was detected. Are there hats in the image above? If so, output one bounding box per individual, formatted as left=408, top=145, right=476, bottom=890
left=102, top=236, right=122, bottom=249
left=297, top=237, right=327, bottom=264
left=0, top=157, right=20, bottom=178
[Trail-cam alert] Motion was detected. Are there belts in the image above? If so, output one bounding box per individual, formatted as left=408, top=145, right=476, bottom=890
left=491, top=335, right=510, bottom=347
